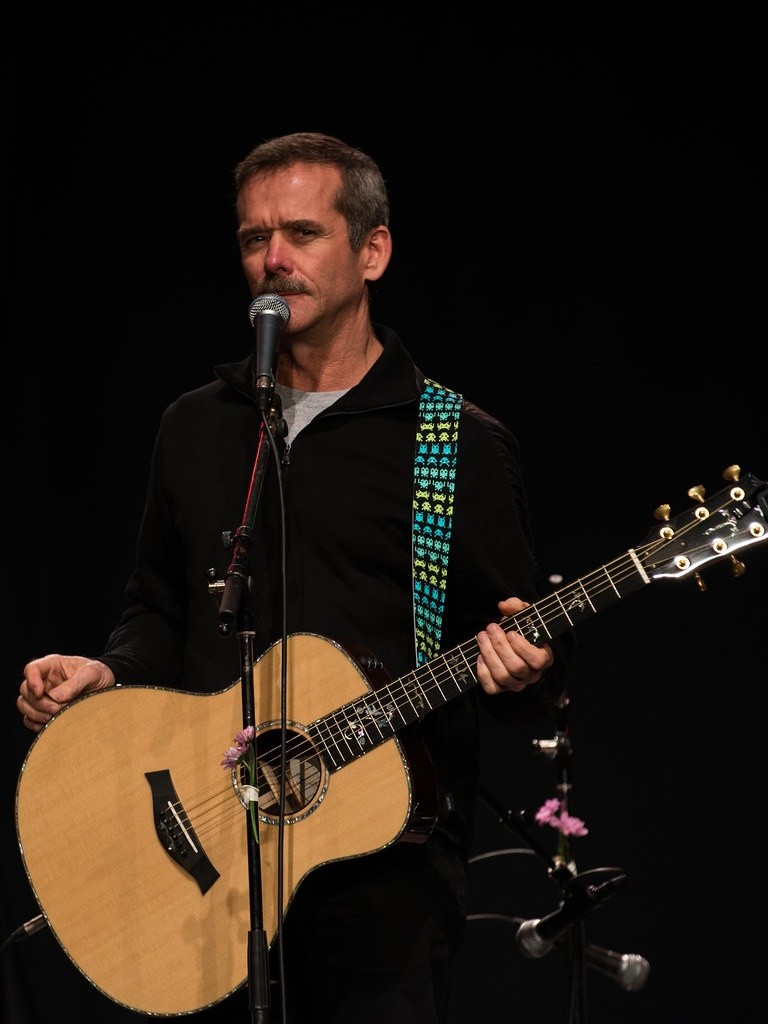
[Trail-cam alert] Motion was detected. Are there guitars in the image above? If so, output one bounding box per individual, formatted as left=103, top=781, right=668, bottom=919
left=14, top=459, right=768, bottom=1019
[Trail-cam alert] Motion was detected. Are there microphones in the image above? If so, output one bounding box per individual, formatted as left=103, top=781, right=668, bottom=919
left=516, top=874, right=629, bottom=958
left=249, top=294, right=291, bottom=412
left=513, top=920, right=651, bottom=991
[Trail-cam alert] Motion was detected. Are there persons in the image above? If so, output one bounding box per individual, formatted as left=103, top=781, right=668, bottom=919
left=15, top=131, right=578, bottom=1022
